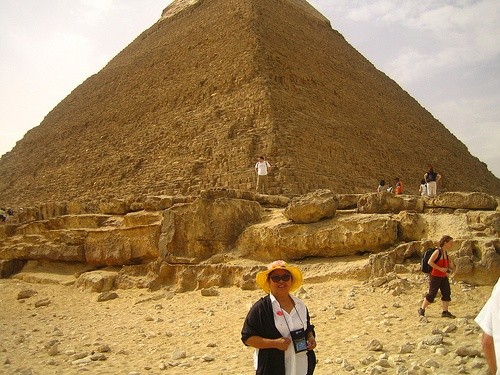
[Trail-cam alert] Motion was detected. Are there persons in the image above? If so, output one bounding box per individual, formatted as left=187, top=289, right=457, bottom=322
left=255, top=156, right=271, bottom=194
left=474, top=276, right=500, bottom=375
left=424, top=167, right=441, bottom=197
left=417, top=235, right=456, bottom=319
left=394, top=178, right=402, bottom=195
left=419, top=179, right=427, bottom=196
left=377, top=180, right=393, bottom=193
left=0, top=207, right=13, bottom=225
left=241, top=260, right=317, bottom=375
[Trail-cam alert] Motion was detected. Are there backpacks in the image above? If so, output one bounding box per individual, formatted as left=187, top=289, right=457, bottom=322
left=421, top=248, right=441, bottom=273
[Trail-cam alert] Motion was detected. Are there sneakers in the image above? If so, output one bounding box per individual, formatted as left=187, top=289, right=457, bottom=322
left=442, top=311, right=453, bottom=317
left=418, top=308, right=425, bottom=316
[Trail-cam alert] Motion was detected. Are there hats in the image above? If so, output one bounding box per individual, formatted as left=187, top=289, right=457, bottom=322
left=256, top=259, right=303, bottom=295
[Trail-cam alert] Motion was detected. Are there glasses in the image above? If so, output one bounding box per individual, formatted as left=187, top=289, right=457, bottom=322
left=267, top=274, right=292, bottom=283
